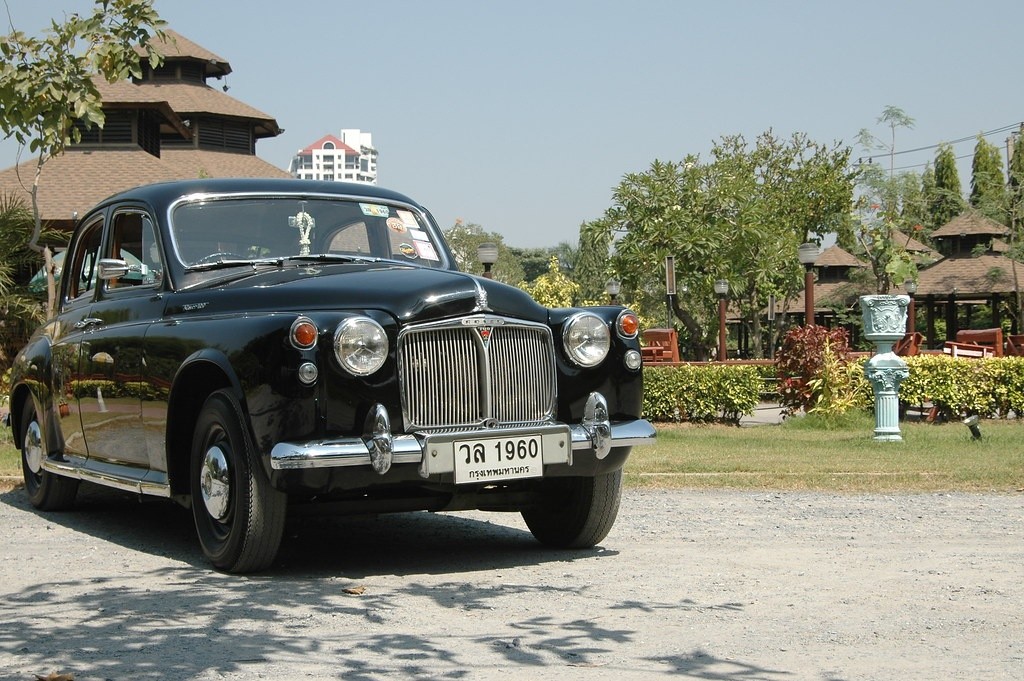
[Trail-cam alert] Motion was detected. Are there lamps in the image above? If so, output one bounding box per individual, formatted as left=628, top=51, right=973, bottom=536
left=963, top=415, right=982, bottom=441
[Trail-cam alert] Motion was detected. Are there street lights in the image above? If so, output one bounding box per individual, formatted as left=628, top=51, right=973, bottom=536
left=798, top=242, right=819, bottom=327
left=713, top=279, right=728, bottom=362
left=905, top=278, right=917, bottom=334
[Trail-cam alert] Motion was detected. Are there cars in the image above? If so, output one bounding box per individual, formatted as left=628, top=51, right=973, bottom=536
left=5, top=178, right=657, bottom=575
